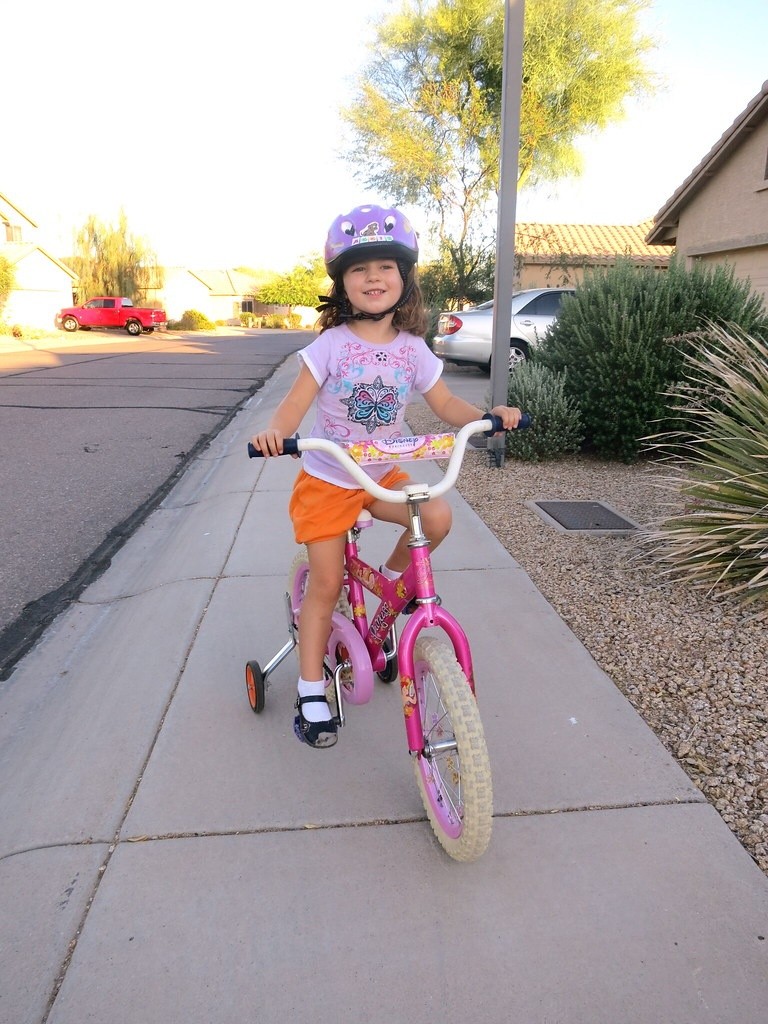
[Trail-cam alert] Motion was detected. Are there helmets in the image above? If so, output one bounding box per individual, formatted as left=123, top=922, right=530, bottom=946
left=325, top=204, right=419, bottom=280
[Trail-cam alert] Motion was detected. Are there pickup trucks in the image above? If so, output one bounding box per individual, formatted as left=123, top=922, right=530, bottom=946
left=57, top=297, right=168, bottom=336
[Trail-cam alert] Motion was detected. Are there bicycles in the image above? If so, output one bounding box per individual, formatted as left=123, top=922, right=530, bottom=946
left=246, top=412, right=531, bottom=862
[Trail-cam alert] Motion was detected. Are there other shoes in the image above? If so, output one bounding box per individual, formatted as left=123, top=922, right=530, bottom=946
left=294, top=691, right=338, bottom=749
left=379, top=564, right=442, bottom=615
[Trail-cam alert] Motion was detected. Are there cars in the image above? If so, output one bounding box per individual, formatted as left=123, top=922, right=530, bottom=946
left=432, top=287, right=591, bottom=376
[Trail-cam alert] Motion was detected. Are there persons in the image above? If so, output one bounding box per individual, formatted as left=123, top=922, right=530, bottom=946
left=249, top=203, right=525, bottom=748
